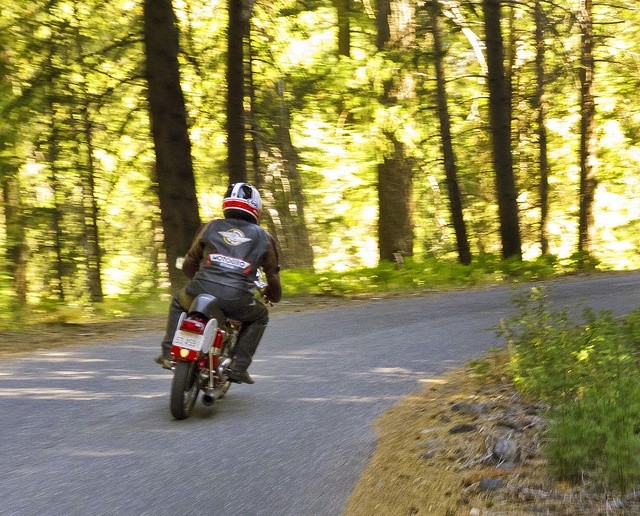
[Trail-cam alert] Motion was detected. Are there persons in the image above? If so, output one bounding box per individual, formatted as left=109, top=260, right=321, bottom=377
left=155, top=182, right=281, bottom=384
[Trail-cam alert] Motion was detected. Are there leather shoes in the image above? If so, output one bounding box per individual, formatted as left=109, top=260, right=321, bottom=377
left=153, top=353, right=173, bottom=364
left=227, top=368, right=254, bottom=384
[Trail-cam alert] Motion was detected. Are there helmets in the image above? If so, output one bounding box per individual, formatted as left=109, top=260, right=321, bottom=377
left=223, top=182, right=262, bottom=225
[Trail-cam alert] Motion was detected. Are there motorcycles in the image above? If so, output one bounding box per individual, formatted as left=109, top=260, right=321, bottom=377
left=161, top=257, right=278, bottom=419
